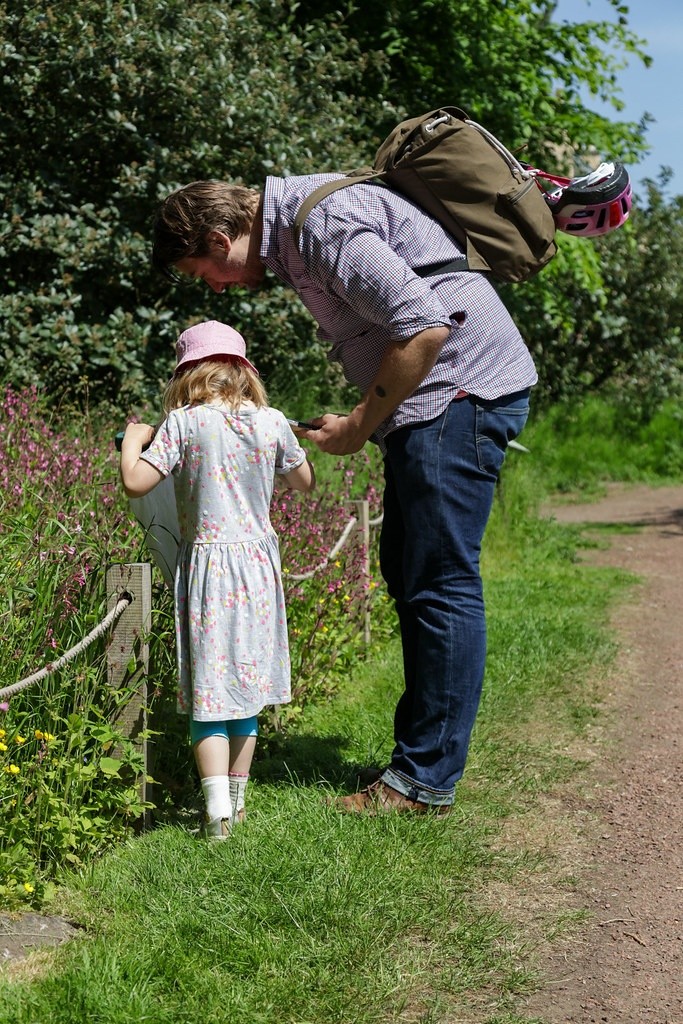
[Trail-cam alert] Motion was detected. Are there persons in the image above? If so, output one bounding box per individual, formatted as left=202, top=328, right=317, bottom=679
left=120, top=321, right=317, bottom=843
left=152, top=172, right=538, bottom=817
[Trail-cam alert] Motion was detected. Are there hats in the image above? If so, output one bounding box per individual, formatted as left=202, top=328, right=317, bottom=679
left=174, top=321, right=259, bottom=376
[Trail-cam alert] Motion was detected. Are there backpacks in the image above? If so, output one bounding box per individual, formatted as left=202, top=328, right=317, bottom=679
left=293, top=105, right=560, bottom=284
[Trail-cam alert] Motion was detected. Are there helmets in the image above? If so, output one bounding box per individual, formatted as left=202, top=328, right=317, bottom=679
left=544, top=160, right=632, bottom=240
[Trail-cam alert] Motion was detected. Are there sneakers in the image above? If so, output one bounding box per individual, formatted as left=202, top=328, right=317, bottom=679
left=323, top=782, right=453, bottom=818
left=192, top=809, right=247, bottom=842
